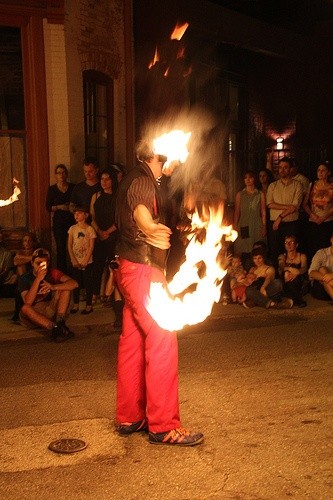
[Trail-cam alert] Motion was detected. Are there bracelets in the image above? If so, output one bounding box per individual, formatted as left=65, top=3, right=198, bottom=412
left=278, top=215, right=283, bottom=219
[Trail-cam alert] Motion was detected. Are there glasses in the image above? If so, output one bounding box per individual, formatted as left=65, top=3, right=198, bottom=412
left=284, top=241, right=297, bottom=245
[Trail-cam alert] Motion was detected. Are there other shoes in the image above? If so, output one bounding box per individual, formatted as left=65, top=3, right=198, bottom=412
left=70, top=304, right=79, bottom=313
left=81, top=308, right=93, bottom=315
left=243, top=301, right=251, bottom=309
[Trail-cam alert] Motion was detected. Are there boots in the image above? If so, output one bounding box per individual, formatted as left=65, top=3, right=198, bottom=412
left=113, top=300, right=124, bottom=327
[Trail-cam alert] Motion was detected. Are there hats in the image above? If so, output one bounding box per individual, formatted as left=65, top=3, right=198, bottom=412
left=32, top=247, right=50, bottom=257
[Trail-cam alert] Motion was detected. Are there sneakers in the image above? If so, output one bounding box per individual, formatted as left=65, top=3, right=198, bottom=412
left=148, top=426, right=204, bottom=445
left=55, top=321, right=74, bottom=337
left=116, top=419, right=148, bottom=433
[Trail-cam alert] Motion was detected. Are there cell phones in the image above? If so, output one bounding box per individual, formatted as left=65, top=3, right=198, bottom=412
left=40, top=260, right=46, bottom=269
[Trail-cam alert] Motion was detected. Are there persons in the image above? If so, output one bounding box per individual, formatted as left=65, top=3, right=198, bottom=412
left=69, top=159, right=101, bottom=305
left=308, top=236, right=333, bottom=301
left=233, top=156, right=333, bottom=269
left=90, top=168, right=116, bottom=305
left=67, top=207, right=97, bottom=315
left=105, top=245, right=123, bottom=327
left=116, top=145, right=204, bottom=446
left=278, top=232, right=308, bottom=307
left=0, top=226, right=36, bottom=299
left=46, top=164, right=75, bottom=275
left=178, top=174, right=226, bottom=222
left=216, top=241, right=282, bottom=309
left=18, top=248, right=78, bottom=338
